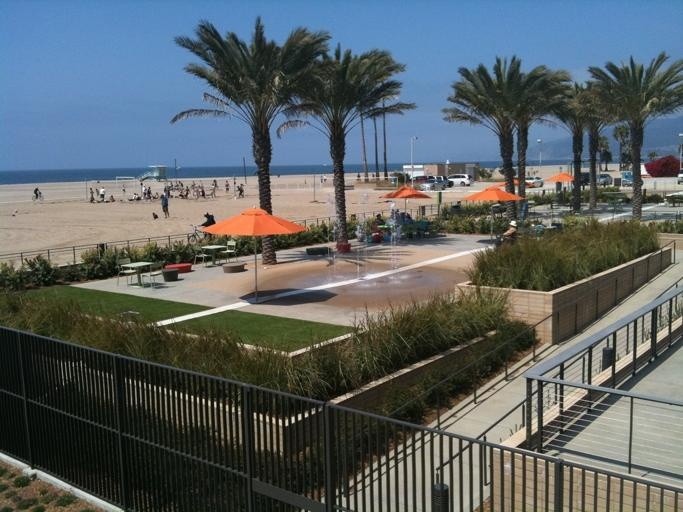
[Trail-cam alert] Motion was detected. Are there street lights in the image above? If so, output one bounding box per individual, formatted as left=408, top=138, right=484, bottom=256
left=410, top=136, right=417, bottom=185
left=536, top=138, right=542, bottom=168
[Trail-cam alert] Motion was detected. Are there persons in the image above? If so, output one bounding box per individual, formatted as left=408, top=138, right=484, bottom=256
left=200, top=212, right=216, bottom=238
left=357, top=172, right=360, bottom=179
left=497, top=220, right=517, bottom=238
left=89, top=179, right=245, bottom=202
left=321, top=175, right=326, bottom=182
left=536, top=220, right=546, bottom=234
left=34, top=186, right=41, bottom=199
left=160, top=195, right=169, bottom=218
left=153, top=213, right=157, bottom=219
left=375, top=207, right=412, bottom=241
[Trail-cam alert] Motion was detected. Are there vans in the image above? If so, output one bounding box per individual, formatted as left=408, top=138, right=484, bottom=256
left=390, top=173, right=404, bottom=184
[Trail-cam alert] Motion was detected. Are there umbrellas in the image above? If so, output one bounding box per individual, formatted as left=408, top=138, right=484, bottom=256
left=203, top=204, right=306, bottom=299
left=379, top=185, right=431, bottom=217
left=496, top=178, right=535, bottom=187
left=545, top=172, right=575, bottom=201
left=462, top=186, right=524, bottom=241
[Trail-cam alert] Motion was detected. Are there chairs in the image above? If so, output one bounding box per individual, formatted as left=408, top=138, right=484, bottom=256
left=116, top=257, right=136, bottom=288
left=216, top=241, right=238, bottom=265
left=191, top=241, right=212, bottom=270
left=139, top=261, right=166, bottom=290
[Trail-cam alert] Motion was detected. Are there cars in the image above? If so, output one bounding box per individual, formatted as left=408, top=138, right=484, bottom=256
left=525, top=176, right=543, bottom=188
left=595, top=174, right=612, bottom=186
left=621, top=171, right=642, bottom=186
left=677, top=170, right=683, bottom=184
left=408, top=173, right=472, bottom=190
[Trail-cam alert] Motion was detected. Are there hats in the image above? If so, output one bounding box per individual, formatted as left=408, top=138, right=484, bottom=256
left=509, top=221, right=518, bottom=227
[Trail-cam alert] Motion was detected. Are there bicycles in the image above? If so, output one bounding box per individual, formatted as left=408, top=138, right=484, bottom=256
left=188, top=224, right=211, bottom=245
left=32, top=191, right=43, bottom=201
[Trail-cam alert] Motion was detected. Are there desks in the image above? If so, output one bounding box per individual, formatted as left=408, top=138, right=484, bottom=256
left=201, top=245, right=227, bottom=268
left=122, top=261, right=154, bottom=288
